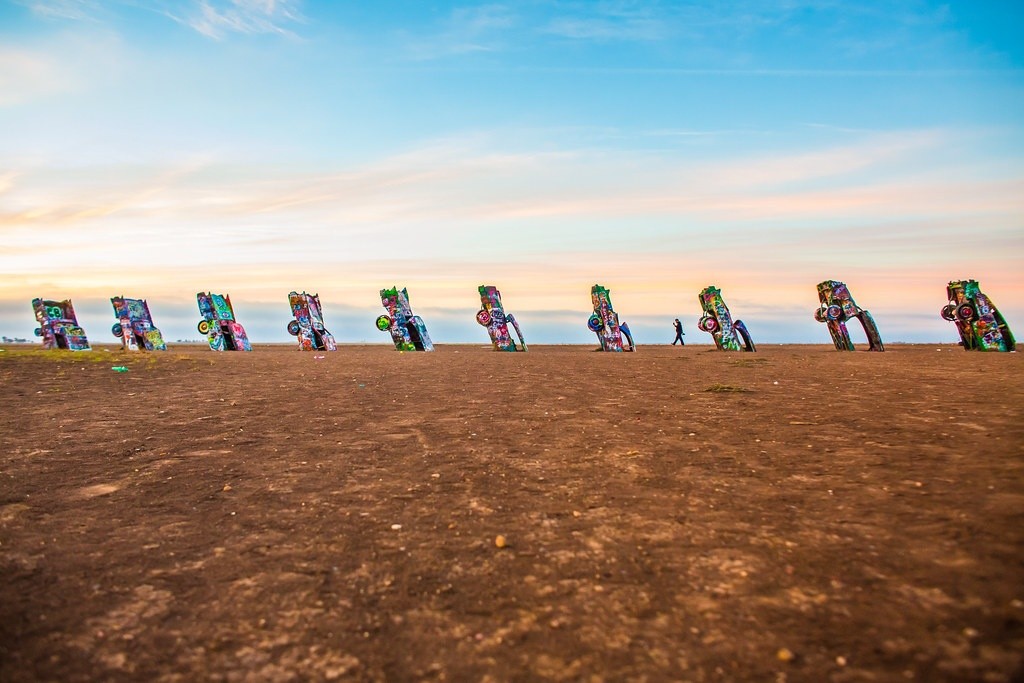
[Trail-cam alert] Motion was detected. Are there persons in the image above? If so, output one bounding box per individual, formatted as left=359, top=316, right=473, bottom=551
left=671, top=319, right=684, bottom=345
